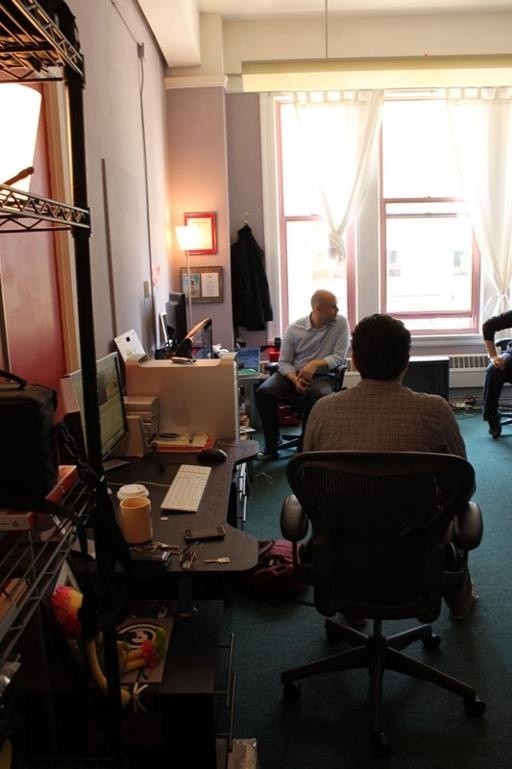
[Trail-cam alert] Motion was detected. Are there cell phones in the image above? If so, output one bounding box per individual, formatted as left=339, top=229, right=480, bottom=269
left=183, top=526, right=226, bottom=542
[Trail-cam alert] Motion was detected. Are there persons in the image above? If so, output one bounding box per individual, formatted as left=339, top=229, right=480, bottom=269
left=482, top=308, right=512, bottom=439
left=303, top=313, right=467, bottom=459
left=255, top=290, right=351, bottom=460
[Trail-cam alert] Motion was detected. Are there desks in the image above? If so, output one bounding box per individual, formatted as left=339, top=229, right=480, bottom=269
left=107, top=437, right=259, bottom=769
left=238, top=373, right=270, bottom=434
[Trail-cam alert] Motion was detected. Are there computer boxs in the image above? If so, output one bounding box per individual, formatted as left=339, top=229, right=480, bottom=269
left=401, top=356, right=450, bottom=401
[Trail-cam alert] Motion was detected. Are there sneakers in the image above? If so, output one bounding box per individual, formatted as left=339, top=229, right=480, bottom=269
left=488, top=410, right=502, bottom=440
left=448, top=589, right=478, bottom=619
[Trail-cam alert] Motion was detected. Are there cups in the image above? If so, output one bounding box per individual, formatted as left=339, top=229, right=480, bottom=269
left=119, top=497, right=154, bottom=545
left=116, top=483, right=150, bottom=501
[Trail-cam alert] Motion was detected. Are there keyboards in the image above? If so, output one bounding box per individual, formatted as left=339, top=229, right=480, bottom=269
left=160, top=463, right=212, bottom=514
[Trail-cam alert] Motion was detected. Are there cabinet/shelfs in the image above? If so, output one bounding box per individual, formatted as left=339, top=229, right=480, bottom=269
left=0, top=0, right=127, bottom=769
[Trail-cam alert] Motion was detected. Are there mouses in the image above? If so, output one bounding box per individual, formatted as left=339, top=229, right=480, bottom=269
left=197, top=448, right=229, bottom=463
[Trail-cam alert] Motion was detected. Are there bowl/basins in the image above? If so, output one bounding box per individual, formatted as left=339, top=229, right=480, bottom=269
left=217, top=351, right=238, bottom=365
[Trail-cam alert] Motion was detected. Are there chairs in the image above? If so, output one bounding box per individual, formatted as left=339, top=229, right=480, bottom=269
left=279, top=449, right=485, bottom=763
left=271, top=362, right=348, bottom=460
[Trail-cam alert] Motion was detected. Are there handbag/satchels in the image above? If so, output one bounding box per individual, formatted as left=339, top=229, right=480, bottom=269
left=235, top=539, right=311, bottom=603
left=0, top=369, right=58, bottom=512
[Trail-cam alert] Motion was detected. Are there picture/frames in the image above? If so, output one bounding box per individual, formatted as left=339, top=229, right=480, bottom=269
left=184, top=212, right=217, bottom=256
left=179, top=266, right=223, bottom=304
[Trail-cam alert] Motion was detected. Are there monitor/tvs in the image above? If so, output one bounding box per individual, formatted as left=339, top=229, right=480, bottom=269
left=180, top=316, right=214, bottom=359
left=61, top=350, right=132, bottom=473
left=165, top=291, right=188, bottom=359
